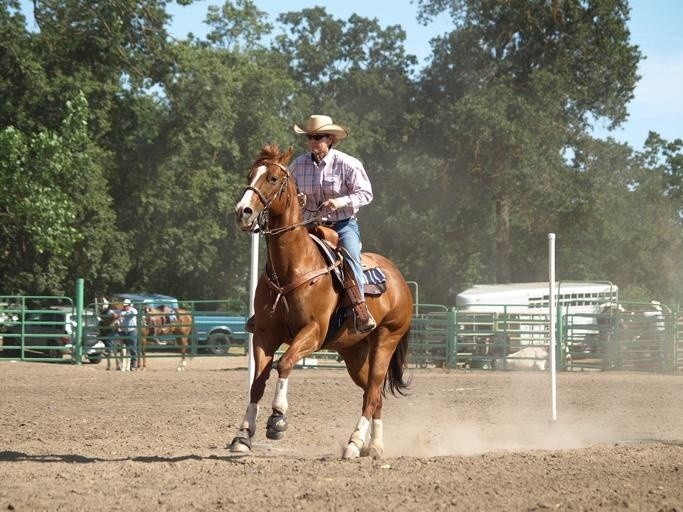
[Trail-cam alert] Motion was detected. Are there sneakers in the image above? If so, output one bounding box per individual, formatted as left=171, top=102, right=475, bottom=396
left=350, top=301, right=377, bottom=334
left=246, top=313, right=258, bottom=333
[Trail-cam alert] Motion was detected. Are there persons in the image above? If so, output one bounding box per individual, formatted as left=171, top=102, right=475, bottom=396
left=597, top=300, right=665, bottom=370
left=245, top=115, right=377, bottom=334
left=117, top=299, right=139, bottom=371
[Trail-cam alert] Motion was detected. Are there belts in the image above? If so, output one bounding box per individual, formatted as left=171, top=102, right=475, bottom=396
left=310, top=217, right=352, bottom=227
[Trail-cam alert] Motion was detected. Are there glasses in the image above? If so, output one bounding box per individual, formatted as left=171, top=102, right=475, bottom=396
left=308, top=134, right=326, bottom=141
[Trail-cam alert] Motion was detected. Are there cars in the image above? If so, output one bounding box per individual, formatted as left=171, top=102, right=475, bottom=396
left=1, top=305, right=102, bottom=364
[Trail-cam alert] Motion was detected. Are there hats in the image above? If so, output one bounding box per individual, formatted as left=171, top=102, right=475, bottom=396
left=294, top=114, right=349, bottom=139
left=123, top=299, right=132, bottom=306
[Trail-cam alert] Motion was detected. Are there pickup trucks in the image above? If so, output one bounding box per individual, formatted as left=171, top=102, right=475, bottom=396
left=102, top=291, right=251, bottom=357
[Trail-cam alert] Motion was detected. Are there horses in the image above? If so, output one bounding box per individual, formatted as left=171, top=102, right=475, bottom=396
left=98, top=303, right=131, bottom=371
left=495, top=346, right=548, bottom=371
left=136, top=308, right=193, bottom=372
left=596, top=306, right=624, bottom=370
left=229, top=142, right=414, bottom=460
